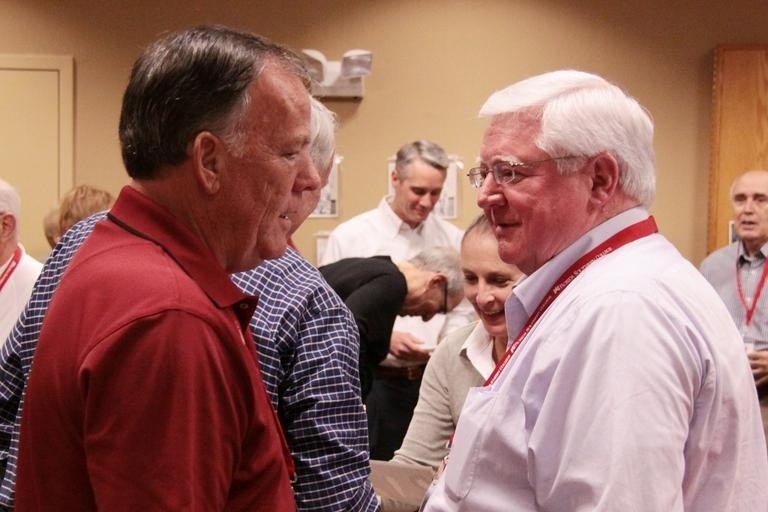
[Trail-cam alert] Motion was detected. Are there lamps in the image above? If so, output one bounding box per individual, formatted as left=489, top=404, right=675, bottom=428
left=297, top=48, right=372, bottom=103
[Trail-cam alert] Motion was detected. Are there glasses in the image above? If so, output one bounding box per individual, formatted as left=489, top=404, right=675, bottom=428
left=438, top=281, right=448, bottom=316
left=466, top=154, right=576, bottom=187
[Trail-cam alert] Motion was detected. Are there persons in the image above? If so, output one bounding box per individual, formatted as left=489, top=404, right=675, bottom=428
left=0, top=96, right=529, bottom=512
left=419, top=66, right=768, bottom=512
left=696, top=174, right=768, bottom=441
left=15, top=23, right=324, bottom=512
